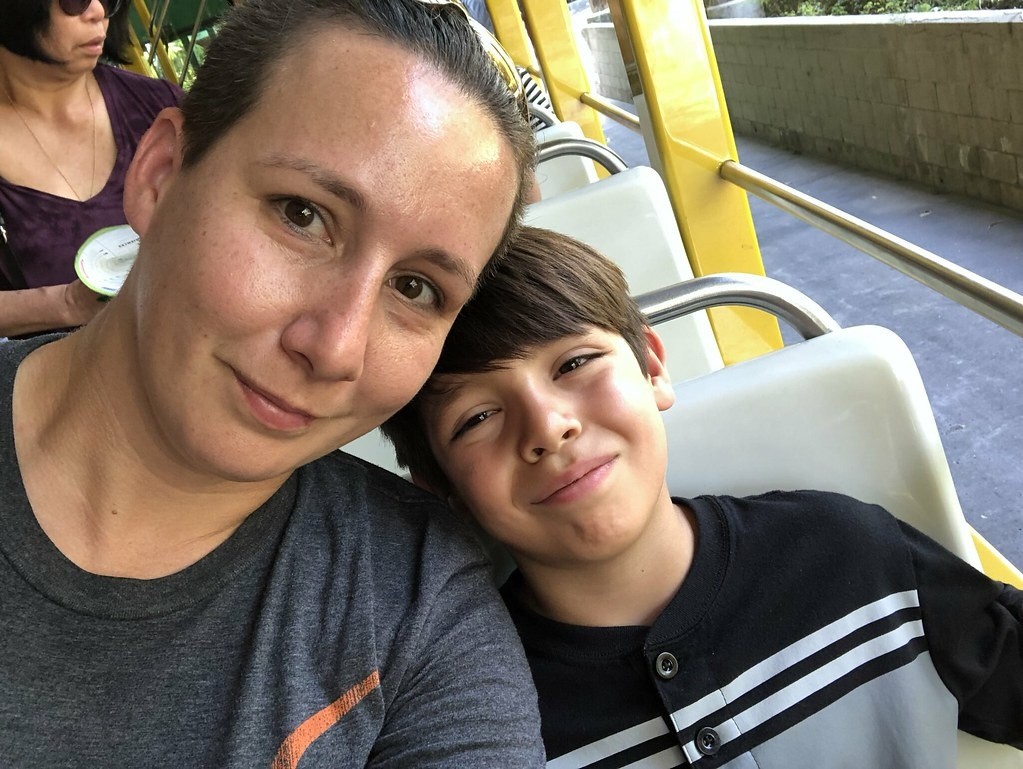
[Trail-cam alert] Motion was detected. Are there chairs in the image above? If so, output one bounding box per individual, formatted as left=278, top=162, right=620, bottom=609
left=332, top=102, right=987, bottom=569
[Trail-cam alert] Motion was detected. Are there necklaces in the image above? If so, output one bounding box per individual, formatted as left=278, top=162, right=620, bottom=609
left=0, top=83, right=96, bottom=202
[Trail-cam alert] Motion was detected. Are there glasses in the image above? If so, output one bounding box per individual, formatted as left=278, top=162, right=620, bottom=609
left=59, top=0, right=122, bottom=18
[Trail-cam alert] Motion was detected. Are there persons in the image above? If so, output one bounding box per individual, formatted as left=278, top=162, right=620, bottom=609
left=0, top=0, right=545, bottom=769
left=379, top=225, right=1023, bottom=769
left=0, top=0, right=188, bottom=339
